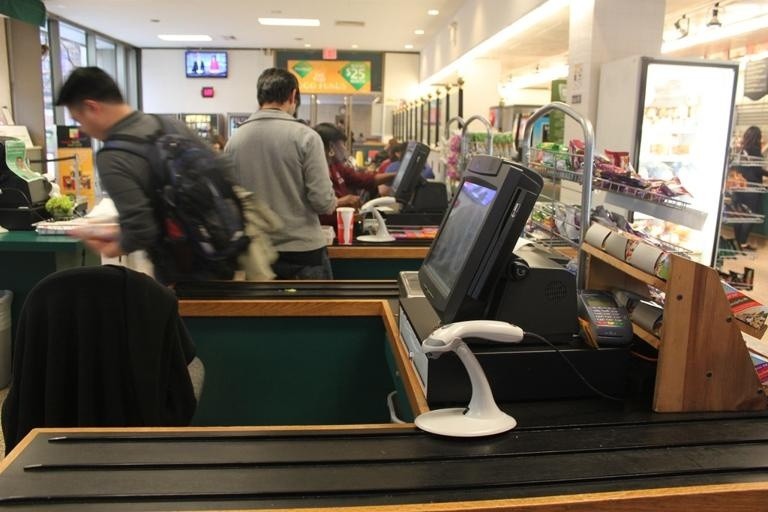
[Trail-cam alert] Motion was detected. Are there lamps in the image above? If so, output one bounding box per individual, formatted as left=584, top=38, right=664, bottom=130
left=706, top=2, right=722, bottom=30
left=672, top=14, right=688, bottom=40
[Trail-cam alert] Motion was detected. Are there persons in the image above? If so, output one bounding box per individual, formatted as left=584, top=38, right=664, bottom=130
left=733, top=126, right=768, bottom=251
left=222, top=67, right=337, bottom=280
left=209, top=55, right=220, bottom=73
left=312, top=119, right=410, bottom=247
left=211, top=139, right=223, bottom=152
left=17, top=156, right=27, bottom=171
left=192, top=53, right=204, bottom=74
left=53, top=66, right=237, bottom=293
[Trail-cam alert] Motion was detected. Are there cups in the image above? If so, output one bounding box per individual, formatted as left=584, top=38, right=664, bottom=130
left=335, top=207, right=355, bottom=246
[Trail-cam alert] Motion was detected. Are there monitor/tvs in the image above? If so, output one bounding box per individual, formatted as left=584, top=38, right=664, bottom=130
left=392, top=141, right=430, bottom=195
left=418, top=154, right=544, bottom=325
left=186, top=51, right=227, bottom=77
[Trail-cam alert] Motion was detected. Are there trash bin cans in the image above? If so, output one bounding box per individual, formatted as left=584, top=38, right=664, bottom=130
left=0, top=289, right=14, bottom=389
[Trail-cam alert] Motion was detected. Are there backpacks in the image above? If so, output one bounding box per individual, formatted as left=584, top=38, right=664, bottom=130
left=97, top=115, right=247, bottom=278
